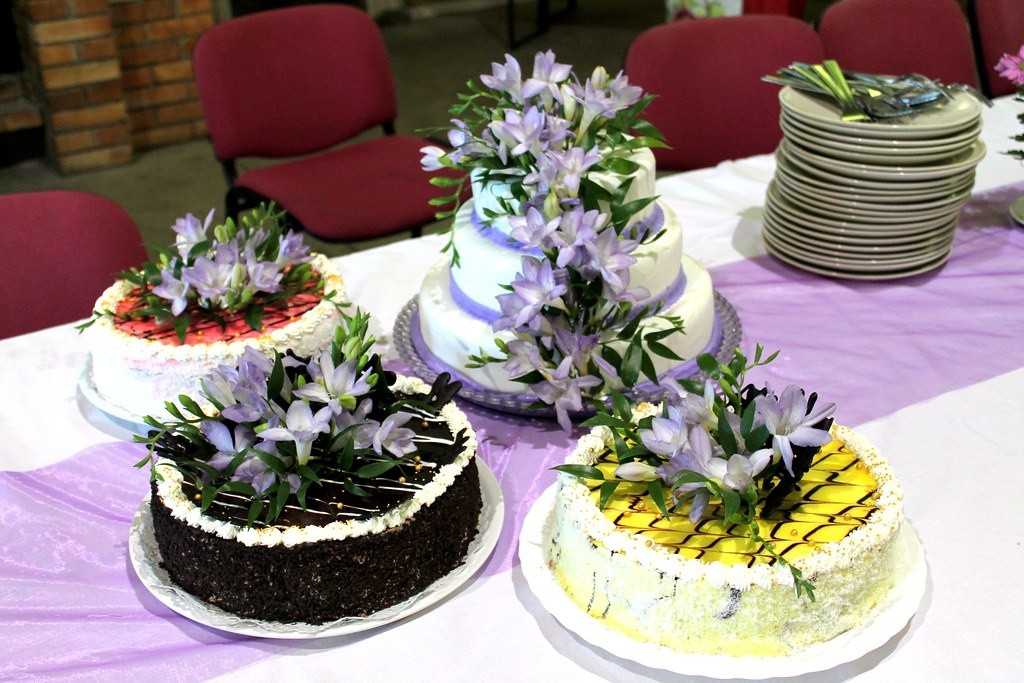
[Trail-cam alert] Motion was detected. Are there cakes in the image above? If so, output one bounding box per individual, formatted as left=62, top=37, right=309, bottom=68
left=129, top=306, right=484, bottom=628
left=417, top=50, right=713, bottom=437
left=544, top=339, right=905, bottom=656
left=76, top=200, right=349, bottom=419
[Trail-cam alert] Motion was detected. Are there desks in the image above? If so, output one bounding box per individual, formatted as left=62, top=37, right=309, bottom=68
left=0, top=92, right=1024, bottom=683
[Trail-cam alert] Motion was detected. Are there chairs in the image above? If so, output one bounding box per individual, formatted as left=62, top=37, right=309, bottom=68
left=192, top=6, right=474, bottom=246
left=625, top=13, right=824, bottom=178
left=0, top=190, right=147, bottom=344
left=976, top=0, right=1024, bottom=99
left=818, top=0, right=982, bottom=96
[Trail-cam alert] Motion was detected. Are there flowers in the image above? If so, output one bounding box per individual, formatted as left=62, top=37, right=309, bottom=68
left=993, top=44, right=1024, bottom=161
left=128, top=306, right=440, bottom=532
left=413, top=50, right=687, bottom=436
left=547, top=342, right=837, bottom=605
left=73, top=200, right=352, bottom=345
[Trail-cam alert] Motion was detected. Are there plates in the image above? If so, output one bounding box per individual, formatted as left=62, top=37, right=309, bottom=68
left=127, top=453, right=509, bottom=637
left=77, top=351, right=188, bottom=429
left=393, top=289, right=743, bottom=414
left=760, top=85, right=985, bottom=281
left=519, top=479, right=928, bottom=679
left=1009, top=196, right=1024, bottom=225
